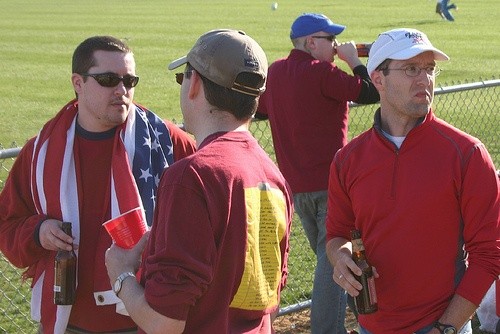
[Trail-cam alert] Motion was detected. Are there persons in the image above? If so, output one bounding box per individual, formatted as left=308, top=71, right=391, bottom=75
left=105, top=29, right=294, bottom=334
left=0, top=36, right=197, bottom=334
left=326, top=28, right=500, bottom=334
left=255, top=13, right=380, bottom=334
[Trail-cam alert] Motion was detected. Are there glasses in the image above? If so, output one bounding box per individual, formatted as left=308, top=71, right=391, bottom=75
left=81, top=73, right=140, bottom=88
left=381, top=66, right=440, bottom=78
left=311, top=35, right=335, bottom=41
left=175, top=71, right=192, bottom=85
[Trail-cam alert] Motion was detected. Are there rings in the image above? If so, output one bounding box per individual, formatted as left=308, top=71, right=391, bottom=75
left=339, top=274, right=343, bottom=279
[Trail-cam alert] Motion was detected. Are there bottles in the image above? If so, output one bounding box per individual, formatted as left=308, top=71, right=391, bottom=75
left=334, top=44, right=372, bottom=57
left=53, top=222, right=77, bottom=305
left=348, top=231, right=378, bottom=316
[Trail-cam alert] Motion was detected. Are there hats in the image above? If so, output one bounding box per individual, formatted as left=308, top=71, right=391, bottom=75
left=289, top=13, right=344, bottom=39
left=168, top=29, right=269, bottom=96
left=367, top=28, right=449, bottom=78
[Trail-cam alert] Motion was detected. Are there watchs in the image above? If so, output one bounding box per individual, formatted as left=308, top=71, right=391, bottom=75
left=434, top=322, right=457, bottom=334
left=112, top=272, right=136, bottom=297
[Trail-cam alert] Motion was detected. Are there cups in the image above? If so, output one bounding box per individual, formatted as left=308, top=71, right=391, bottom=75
left=102, top=206, right=147, bottom=249
